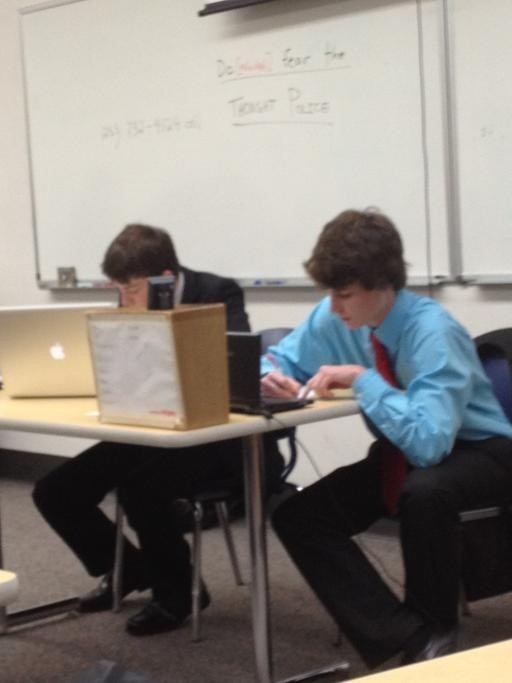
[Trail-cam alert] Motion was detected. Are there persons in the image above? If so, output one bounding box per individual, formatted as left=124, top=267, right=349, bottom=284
left=29, top=222, right=301, bottom=637
left=255, top=203, right=512, bottom=672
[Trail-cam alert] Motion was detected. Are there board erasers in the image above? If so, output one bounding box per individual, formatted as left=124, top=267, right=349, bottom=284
left=57, top=267, right=75, bottom=287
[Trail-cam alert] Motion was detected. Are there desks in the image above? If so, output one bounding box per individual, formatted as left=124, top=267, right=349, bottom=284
left=339, top=637, right=511, bottom=682
left=0, top=387, right=361, bottom=682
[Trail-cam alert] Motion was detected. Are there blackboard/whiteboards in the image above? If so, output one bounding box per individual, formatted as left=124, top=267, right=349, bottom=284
left=16, top=1, right=454, bottom=290
left=445, top=4, right=511, bottom=291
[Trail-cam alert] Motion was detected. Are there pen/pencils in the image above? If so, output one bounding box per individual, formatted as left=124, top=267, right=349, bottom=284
left=267, top=352, right=284, bottom=374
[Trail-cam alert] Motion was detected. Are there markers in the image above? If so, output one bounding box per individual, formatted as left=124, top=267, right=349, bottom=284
left=254, top=279, right=288, bottom=284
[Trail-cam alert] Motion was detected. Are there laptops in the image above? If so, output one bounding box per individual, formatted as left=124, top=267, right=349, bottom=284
left=226, top=331, right=315, bottom=415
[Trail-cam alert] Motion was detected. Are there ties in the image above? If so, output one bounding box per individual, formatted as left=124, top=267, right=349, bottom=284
left=368, top=334, right=406, bottom=515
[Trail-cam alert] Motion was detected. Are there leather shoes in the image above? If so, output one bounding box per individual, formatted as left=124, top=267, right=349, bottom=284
left=400, top=629, right=458, bottom=668
left=76, top=564, right=210, bottom=636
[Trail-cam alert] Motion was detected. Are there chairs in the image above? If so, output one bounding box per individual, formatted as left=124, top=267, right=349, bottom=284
left=334, top=327, right=512, bottom=647
left=111, top=329, right=300, bottom=642
